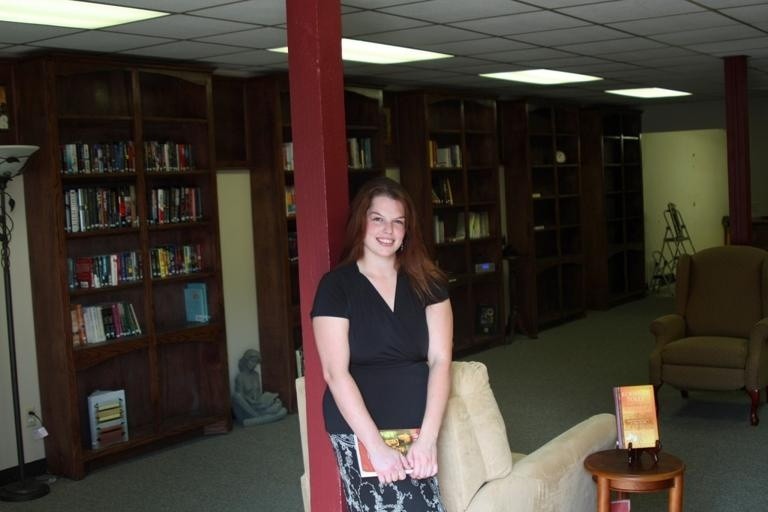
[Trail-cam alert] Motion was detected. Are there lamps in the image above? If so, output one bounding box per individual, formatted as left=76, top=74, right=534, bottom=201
left=0, top=144, right=50, bottom=503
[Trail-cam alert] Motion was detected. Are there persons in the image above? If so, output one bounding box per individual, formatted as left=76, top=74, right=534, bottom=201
left=309, top=176, right=453, bottom=512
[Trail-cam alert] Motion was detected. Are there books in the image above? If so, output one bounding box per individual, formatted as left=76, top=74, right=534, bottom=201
left=282, top=139, right=494, bottom=284
left=613, top=384, right=659, bottom=451
left=61, top=139, right=206, bottom=290
left=353, top=428, right=421, bottom=478
left=70, top=302, right=140, bottom=345
left=183, top=281, right=210, bottom=322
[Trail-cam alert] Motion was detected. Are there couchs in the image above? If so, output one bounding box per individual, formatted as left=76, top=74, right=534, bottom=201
left=650, top=245, right=768, bottom=427
left=293, top=361, right=620, bottom=512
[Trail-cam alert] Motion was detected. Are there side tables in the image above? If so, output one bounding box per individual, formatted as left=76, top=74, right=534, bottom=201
left=584, top=448, right=686, bottom=512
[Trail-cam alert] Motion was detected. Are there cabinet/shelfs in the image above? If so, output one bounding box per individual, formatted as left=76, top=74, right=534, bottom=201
left=391, top=89, right=508, bottom=362
left=247, top=74, right=385, bottom=417
left=12, top=48, right=231, bottom=481
left=586, top=103, right=644, bottom=313
left=507, top=98, right=585, bottom=339
